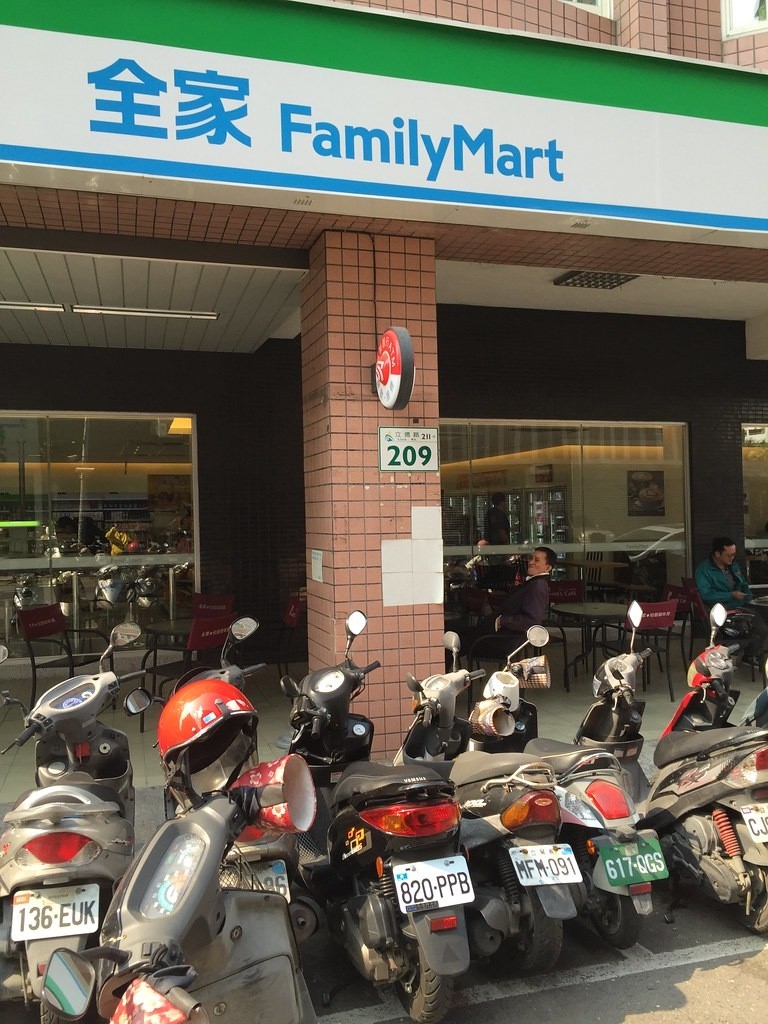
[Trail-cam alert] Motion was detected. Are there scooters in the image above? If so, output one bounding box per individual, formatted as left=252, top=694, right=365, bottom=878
left=386, top=630, right=588, bottom=978
left=659, top=602, right=767, bottom=741
left=36, top=733, right=318, bottom=1023
left=0, top=624, right=152, bottom=1024
left=474, top=625, right=671, bottom=953
left=9, top=532, right=192, bottom=625
left=278, top=610, right=475, bottom=1024
left=577, top=601, right=768, bottom=939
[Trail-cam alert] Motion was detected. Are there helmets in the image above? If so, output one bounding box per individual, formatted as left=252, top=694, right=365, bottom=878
left=127, top=541, right=142, bottom=553
left=157, top=678, right=258, bottom=809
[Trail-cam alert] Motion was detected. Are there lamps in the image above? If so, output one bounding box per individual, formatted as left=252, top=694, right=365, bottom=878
left=72, top=304, right=221, bottom=319
left=0, top=301, right=65, bottom=312
left=555, top=271, right=639, bottom=289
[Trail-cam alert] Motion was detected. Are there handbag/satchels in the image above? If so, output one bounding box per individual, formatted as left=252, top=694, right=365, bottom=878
left=722, top=617, right=752, bottom=640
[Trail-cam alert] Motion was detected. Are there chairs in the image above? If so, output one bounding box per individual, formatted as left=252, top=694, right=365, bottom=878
left=190, top=583, right=309, bottom=680
left=445, top=557, right=768, bottom=703
left=17, top=602, right=118, bottom=714
left=140, top=611, right=241, bottom=732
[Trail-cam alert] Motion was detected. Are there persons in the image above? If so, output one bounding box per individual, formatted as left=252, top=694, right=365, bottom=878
left=58, top=517, right=110, bottom=552
left=484, top=492, right=511, bottom=564
left=695, top=537, right=765, bottom=670
left=446, top=547, right=557, bottom=673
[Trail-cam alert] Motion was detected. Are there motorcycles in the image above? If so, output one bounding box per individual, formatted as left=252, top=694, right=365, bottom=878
left=123, top=614, right=318, bottom=948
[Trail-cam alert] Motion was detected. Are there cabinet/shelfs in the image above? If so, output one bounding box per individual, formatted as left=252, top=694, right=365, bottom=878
left=1, top=494, right=151, bottom=548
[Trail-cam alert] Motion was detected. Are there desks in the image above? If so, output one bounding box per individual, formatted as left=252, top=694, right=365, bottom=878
left=142, top=618, right=223, bottom=696
left=551, top=559, right=629, bottom=581
left=587, top=581, right=659, bottom=640
left=745, top=595, right=768, bottom=608
left=549, top=601, right=631, bottom=691
